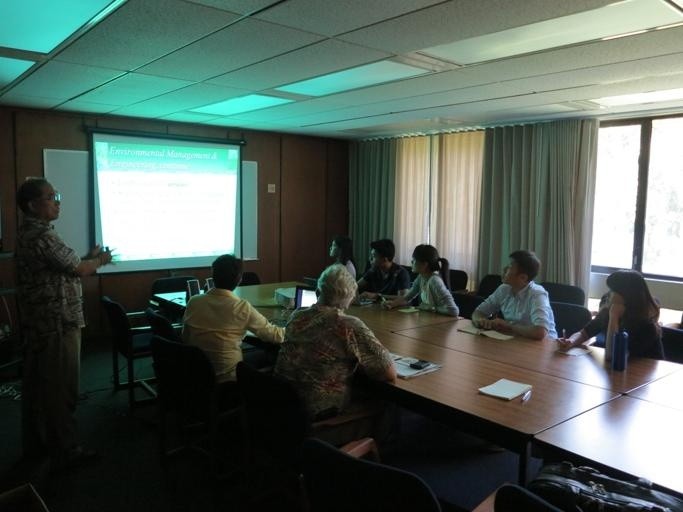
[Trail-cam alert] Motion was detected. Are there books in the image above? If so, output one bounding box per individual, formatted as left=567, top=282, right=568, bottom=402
left=390, top=353, right=441, bottom=380
left=457, top=324, right=514, bottom=340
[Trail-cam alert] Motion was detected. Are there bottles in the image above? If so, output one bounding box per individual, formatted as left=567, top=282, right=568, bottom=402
left=611, top=327, right=629, bottom=372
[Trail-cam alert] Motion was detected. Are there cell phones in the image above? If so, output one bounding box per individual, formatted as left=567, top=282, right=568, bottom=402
left=409, top=358, right=430, bottom=370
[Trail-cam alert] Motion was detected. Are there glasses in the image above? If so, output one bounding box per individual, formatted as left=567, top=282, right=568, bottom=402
left=34, top=192, right=63, bottom=203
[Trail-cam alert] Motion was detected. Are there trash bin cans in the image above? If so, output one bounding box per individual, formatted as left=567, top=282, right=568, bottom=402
left=0, top=482, right=50, bottom=512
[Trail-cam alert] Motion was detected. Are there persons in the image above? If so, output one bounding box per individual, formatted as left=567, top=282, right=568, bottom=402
left=350, top=239, right=411, bottom=304
left=271, top=263, right=405, bottom=470
left=14, top=178, right=120, bottom=466
left=381, top=244, right=460, bottom=316
left=182, top=255, right=286, bottom=428
left=556, top=270, right=666, bottom=360
left=329, top=236, right=356, bottom=281
left=471, top=249, right=558, bottom=340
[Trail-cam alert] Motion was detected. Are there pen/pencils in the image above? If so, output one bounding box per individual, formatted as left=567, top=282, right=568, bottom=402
left=522, top=391, right=532, bottom=401
left=378, top=293, right=386, bottom=303
left=394, top=357, right=402, bottom=361
left=562, top=329, right=566, bottom=342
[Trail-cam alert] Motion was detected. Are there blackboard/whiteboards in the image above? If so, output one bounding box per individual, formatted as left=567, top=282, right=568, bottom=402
left=43, top=148, right=258, bottom=261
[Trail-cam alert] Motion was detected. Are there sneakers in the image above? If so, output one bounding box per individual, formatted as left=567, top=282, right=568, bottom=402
left=23, top=443, right=103, bottom=475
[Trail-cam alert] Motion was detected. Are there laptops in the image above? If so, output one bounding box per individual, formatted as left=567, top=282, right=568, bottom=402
left=295, top=284, right=318, bottom=310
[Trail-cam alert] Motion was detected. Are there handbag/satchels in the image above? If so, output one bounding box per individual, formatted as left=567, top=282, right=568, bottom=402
left=525, top=459, right=683, bottom=512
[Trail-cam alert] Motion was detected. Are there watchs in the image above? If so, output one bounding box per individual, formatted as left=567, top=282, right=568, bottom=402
left=506, top=320, right=516, bottom=335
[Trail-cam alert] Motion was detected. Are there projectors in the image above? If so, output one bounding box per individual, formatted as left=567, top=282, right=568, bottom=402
left=273, top=287, right=296, bottom=310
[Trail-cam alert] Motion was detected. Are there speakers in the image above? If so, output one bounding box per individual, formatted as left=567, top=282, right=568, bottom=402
left=186, top=279, right=201, bottom=303
left=203, top=277, right=214, bottom=292
